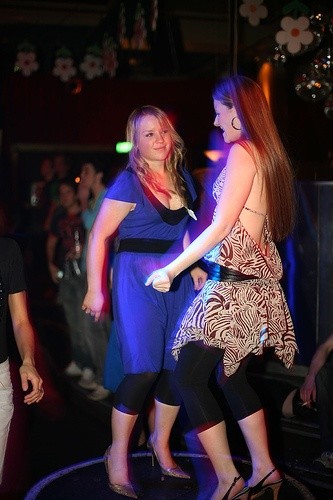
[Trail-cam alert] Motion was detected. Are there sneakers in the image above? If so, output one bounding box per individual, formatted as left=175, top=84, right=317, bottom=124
left=312, top=451, right=333, bottom=469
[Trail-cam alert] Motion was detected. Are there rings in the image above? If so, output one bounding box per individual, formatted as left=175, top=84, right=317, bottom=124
left=39, top=390, right=43, bottom=394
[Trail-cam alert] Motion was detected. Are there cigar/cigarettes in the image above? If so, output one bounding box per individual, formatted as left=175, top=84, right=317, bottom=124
left=300, top=400, right=312, bottom=407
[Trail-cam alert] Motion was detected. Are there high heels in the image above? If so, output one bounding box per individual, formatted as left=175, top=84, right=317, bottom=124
left=103, top=444, right=140, bottom=499
left=220, top=472, right=251, bottom=500
left=248, top=469, right=283, bottom=500
left=146, top=434, right=193, bottom=480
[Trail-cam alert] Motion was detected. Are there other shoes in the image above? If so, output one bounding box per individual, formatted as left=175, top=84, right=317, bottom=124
left=66, top=360, right=110, bottom=402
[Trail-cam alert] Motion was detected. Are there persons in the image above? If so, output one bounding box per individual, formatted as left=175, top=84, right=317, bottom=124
left=21, top=154, right=125, bottom=402
left=144, top=76, right=295, bottom=500
left=80, top=106, right=192, bottom=499
left=0, top=236, right=45, bottom=488
left=301, top=334, right=333, bottom=473
